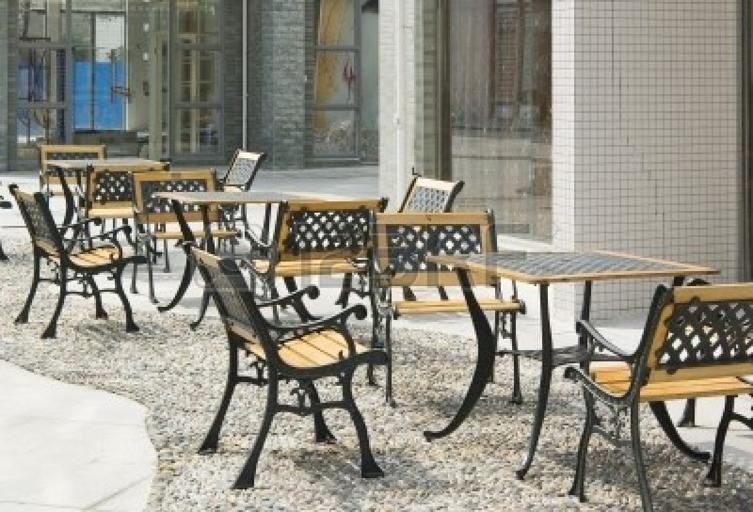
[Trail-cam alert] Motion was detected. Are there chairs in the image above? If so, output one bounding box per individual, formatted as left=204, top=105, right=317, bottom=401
left=367, top=207, right=529, bottom=411
left=240, top=197, right=390, bottom=327
left=562, top=282, right=753, bottom=512
left=7, top=182, right=148, bottom=341
left=172, top=148, right=270, bottom=249
left=333, top=165, right=466, bottom=309
left=72, top=162, right=174, bottom=275
left=127, top=168, right=242, bottom=305
left=178, top=238, right=393, bottom=492
left=34, top=142, right=109, bottom=242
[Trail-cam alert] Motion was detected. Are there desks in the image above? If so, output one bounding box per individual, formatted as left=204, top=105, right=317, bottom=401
left=151, top=189, right=339, bottom=331
left=43, top=156, right=164, bottom=257
left=421, top=247, right=723, bottom=464
